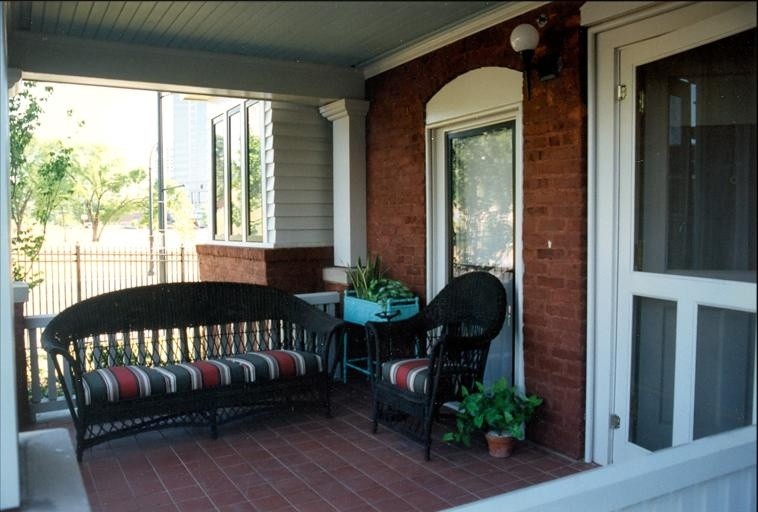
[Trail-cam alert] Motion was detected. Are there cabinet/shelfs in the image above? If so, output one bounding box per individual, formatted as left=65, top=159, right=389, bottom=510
left=343, top=289, right=421, bottom=385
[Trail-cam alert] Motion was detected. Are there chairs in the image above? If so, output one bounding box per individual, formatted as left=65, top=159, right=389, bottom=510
left=364, top=272, right=507, bottom=464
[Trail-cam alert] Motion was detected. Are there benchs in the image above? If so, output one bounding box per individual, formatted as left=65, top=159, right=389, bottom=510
left=41, top=282, right=350, bottom=462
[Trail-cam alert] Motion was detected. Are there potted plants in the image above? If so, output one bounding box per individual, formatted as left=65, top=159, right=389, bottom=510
left=441, top=377, right=545, bottom=457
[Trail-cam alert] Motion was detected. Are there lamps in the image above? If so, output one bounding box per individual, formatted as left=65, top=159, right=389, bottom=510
left=509, top=23, right=562, bottom=101
